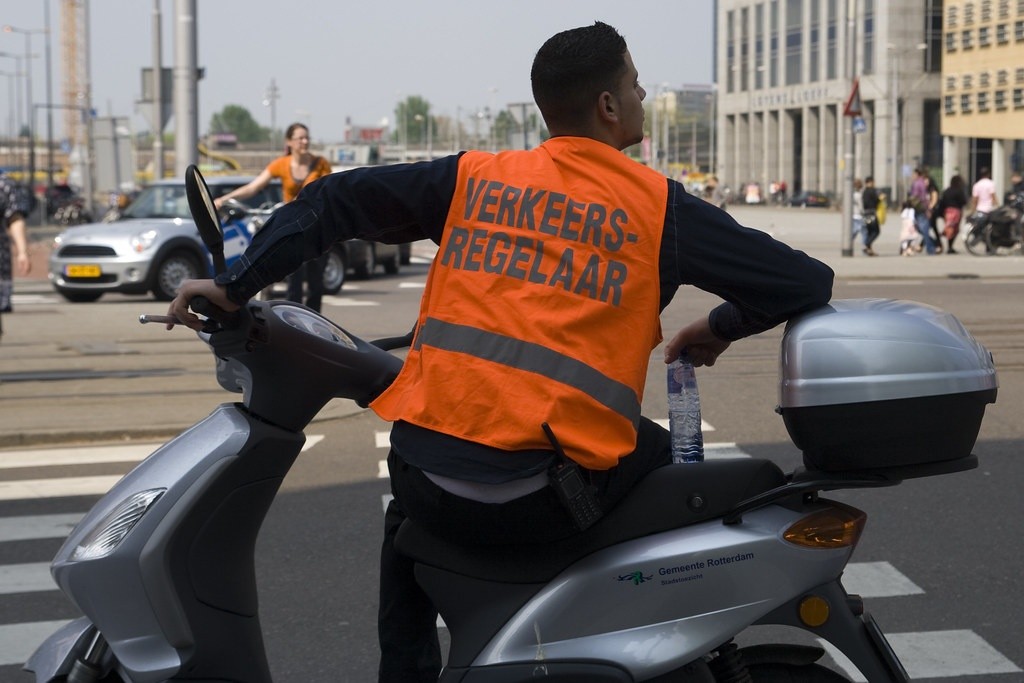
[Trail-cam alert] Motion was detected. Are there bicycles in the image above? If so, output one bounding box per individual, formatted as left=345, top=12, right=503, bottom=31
left=963, top=195, right=1024, bottom=260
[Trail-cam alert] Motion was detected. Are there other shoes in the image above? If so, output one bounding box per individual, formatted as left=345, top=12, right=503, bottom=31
left=862, top=246, right=873, bottom=255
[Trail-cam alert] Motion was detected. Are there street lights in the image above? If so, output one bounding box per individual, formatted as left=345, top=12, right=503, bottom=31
left=0, top=26, right=53, bottom=143
left=413, top=114, right=427, bottom=153
left=887, top=43, right=930, bottom=212
left=0, top=50, right=42, bottom=166
left=650, top=80, right=671, bottom=169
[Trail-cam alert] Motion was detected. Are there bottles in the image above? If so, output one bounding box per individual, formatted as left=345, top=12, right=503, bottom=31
left=667, top=353, right=704, bottom=461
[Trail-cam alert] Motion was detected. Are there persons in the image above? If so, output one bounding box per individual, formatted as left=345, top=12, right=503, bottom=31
left=963, top=166, right=1024, bottom=253
left=851, top=176, right=881, bottom=258
left=900, top=165, right=969, bottom=257
left=166, top=20, right=834, bottom=683
left=213, top=123, right=331, bottom=314
left=0, top=172, right=31, bottom=349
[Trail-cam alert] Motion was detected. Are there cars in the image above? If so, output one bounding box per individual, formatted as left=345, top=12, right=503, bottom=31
left=330, top=165, right=412, bottom=280
left=44, top=173, right=351, bottom=305
left=786, top=190, right=830, bottom=209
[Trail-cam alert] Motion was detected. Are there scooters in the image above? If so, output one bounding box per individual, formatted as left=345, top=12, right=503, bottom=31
left=18, top=160, right=1003, bottom=683
